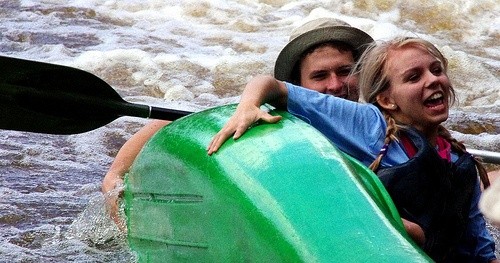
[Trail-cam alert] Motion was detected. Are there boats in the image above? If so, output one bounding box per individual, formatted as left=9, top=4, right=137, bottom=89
left=125, top=92, right=440, bottom=262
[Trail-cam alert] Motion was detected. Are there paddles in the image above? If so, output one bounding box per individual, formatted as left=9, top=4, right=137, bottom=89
left=1, top=56, right=500, bottom=166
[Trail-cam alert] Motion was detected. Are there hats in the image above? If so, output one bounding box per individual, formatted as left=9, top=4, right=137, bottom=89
left=274, top=18, right=376, bottom=81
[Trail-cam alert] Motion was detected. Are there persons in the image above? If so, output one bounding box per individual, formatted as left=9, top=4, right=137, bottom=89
left=206, top=34, right=499, bottom=263
left=103, top=15, right=376, bottom=239
